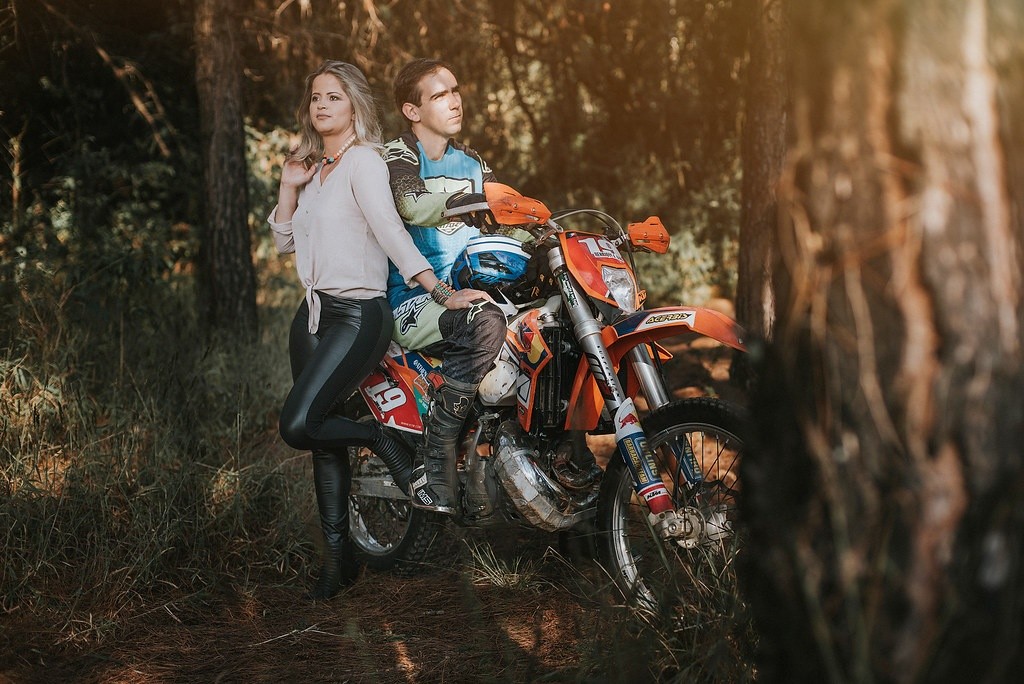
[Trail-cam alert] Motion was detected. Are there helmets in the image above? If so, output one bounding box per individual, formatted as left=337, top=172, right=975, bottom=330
left=446, top=234, right=532, bottom=291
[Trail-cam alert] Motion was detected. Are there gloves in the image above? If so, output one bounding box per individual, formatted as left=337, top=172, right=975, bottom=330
left=485, top=241, right=559, bottom=304
left=446, top=192, right=501, bottom=235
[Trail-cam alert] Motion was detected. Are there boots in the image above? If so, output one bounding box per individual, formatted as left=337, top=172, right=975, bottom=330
left=549, top=447, right=652, bottom=578
left=409, top=370, right=480, bottom=517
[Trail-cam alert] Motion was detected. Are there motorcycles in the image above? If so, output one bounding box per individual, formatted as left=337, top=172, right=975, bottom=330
left=344, top=181, right=798, bottom=638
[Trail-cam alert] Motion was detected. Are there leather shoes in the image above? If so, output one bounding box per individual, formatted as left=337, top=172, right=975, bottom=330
left=362, top=420, right=417, bottom=496
left=307, top=534, right=350, bottom=598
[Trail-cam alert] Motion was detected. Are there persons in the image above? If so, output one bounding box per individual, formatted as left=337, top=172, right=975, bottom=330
left=379, top=61, right=559, bottom=505
left=268, top=60, right=497, bottom=598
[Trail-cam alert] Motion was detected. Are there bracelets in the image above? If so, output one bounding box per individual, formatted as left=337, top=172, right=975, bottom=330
left=430, top=279, right=457, bottom=306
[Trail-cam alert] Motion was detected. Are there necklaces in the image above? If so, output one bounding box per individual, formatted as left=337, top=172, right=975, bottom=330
left=322, top=134, right=356, bottom=165
